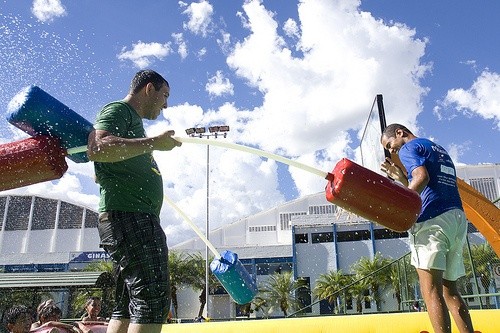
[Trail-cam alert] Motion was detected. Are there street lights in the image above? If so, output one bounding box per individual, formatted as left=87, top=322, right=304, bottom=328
left=185, top=125, right=230, bottom=318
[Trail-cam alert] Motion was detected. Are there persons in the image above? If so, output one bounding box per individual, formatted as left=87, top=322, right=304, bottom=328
left=82, top=297, right=107, bottom=325
left=3, top=304, right=61, bottom=333
left=29, top=299, right=94, bottom=333
left=87, top=69, right=182, bottom=333
left=380, top=123, right=475, bottom=333
